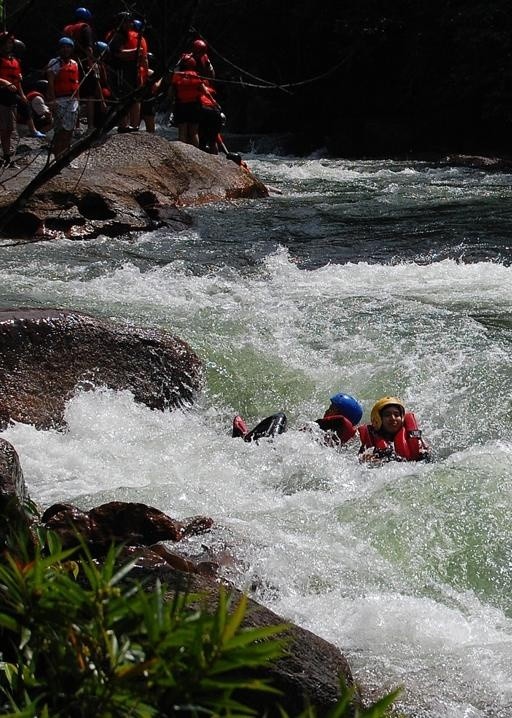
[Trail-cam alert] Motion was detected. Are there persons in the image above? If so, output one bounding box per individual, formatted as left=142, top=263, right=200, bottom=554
left=233, top=394, right=363, bottom=444
left=357, top=395, right=431, bottom=465
left=1, top=7, right=248, bottom=169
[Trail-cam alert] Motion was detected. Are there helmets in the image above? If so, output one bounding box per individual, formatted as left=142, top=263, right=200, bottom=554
left=370, top=396, right=406, bottom=428
left=330, top=393, right=363, bottom=427
left=1, top=6, right=208, bottom=98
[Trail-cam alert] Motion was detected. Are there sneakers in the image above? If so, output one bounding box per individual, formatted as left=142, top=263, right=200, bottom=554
left=32, top=130, right=47, bottom=139
